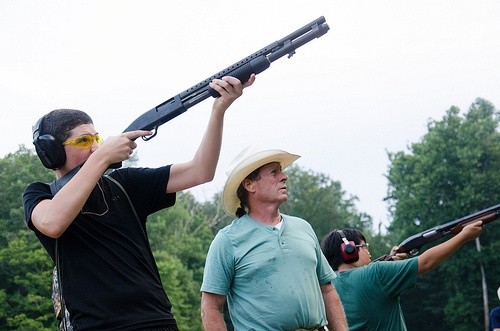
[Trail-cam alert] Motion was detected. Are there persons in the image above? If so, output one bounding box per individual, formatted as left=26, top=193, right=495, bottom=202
left=489, top=308, right=500, bottom=331
left=201, top=160, right=353, bottom=331
left=322, top=221, right=487, bottom=331
left=23, top=72, right=256, bottom=330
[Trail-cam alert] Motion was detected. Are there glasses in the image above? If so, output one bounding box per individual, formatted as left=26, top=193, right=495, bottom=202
left=356, top=243, right=369, bottom=251
left=62, top=133, right=103, bottom=148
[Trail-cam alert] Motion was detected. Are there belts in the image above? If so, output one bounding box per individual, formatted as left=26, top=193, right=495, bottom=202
left=294, top=326, right=327, bottom=331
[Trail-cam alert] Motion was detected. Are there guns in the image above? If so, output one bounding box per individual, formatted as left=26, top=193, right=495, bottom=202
left=48, top=14, right=331, bottom=198
left=370, top=204, right=500, bottom=263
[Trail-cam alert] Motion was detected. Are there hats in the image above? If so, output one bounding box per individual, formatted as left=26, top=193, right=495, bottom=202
left=222, top=145, right=302, bottom=218
